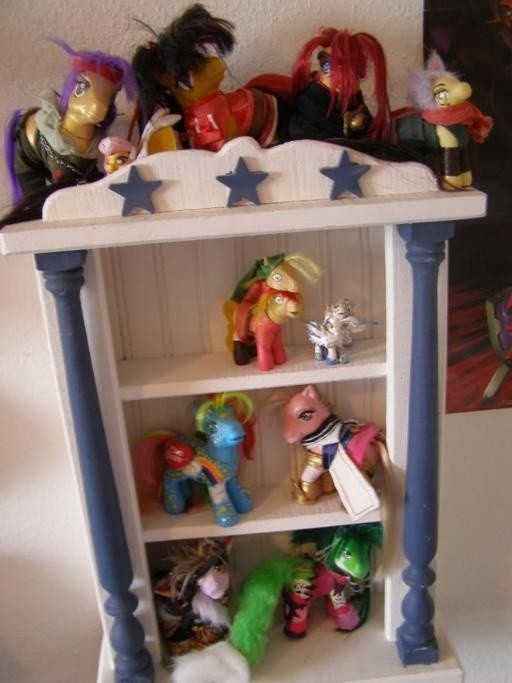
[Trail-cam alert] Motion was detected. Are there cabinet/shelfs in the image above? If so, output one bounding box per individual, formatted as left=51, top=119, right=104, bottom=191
left=0, top=136, right=489, bottom=683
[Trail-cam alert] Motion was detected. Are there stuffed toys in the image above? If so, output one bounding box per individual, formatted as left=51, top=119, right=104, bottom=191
left=167, top=551, right=315, bottom=683
